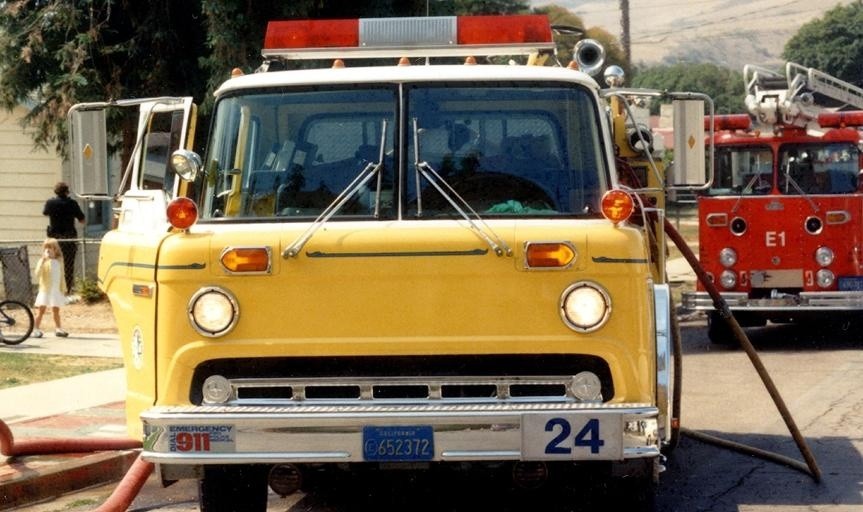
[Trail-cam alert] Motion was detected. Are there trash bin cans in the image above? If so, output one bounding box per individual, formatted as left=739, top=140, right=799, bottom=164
left=1, top=245, right=34, bottom=310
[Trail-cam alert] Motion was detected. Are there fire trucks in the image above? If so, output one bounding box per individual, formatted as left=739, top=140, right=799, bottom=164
left=681, top=62, right=861, bottom=348
left=68, top=14, right=713, bottom=512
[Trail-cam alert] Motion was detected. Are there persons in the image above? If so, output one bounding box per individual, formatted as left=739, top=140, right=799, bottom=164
left=31, top=238, right=70, bottom=340
left=42, top=181, right=86, bottom=296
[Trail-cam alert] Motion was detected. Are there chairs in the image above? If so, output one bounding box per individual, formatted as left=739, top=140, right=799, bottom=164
left=481, top=136, right=571, bottom=213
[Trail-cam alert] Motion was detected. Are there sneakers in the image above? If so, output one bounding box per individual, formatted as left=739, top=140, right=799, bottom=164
left=56, top=330, right=68, bottom=337
left=30, top=328, right=43, bottom=337
